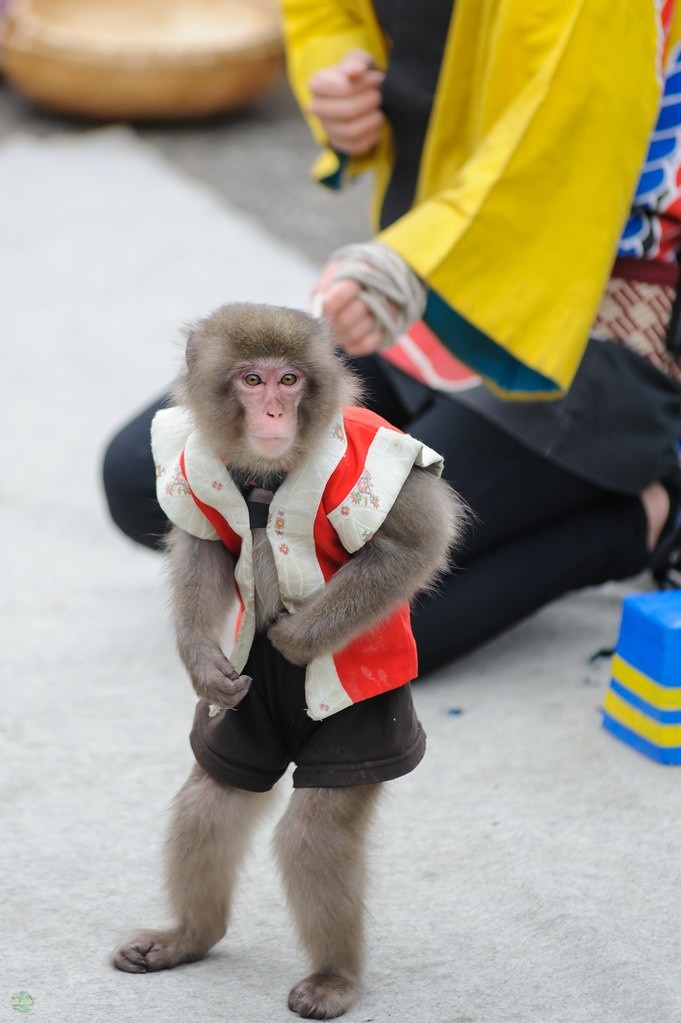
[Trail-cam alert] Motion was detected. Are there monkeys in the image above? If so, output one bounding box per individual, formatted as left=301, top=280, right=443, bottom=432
left=111, top=302, right=477, bottom=1022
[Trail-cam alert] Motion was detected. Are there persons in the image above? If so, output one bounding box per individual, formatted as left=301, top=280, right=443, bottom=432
left=101, top=0, right=681, bottom=686
